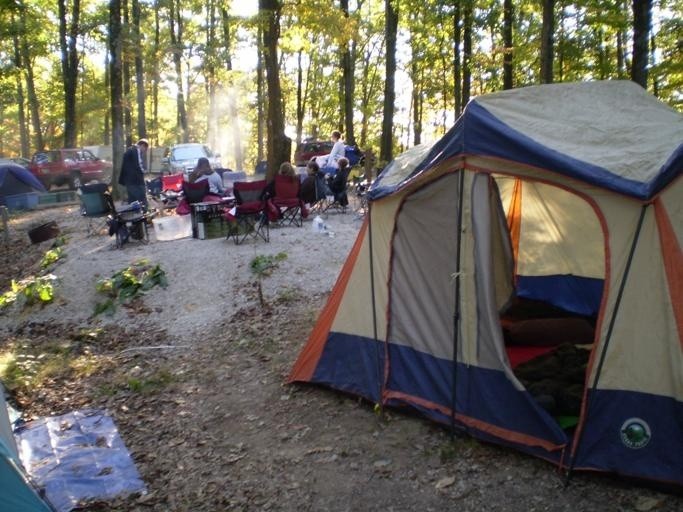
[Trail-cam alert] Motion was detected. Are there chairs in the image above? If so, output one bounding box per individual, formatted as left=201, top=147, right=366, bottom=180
left=106, top=166, right=357, bottom=246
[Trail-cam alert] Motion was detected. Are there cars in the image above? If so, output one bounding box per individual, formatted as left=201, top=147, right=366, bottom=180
left=160, top=144, right=224, bottom=176
left=294, top=141, right=334, bottom=166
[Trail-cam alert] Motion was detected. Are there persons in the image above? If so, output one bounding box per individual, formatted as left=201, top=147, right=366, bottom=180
left=260, top=162, right=301, bottom=202
left=189, top=158, right=224, bottom=196
left=299, top=162, right=325, bottom=204
left=323, top=131, right=345, bottom=169
left=325, top=159, right=348, bottom=195
left=119, top=139, right=149, bottom=214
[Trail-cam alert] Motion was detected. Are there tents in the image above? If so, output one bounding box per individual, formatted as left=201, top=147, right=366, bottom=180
left=286, top=80, right=683, bottom=484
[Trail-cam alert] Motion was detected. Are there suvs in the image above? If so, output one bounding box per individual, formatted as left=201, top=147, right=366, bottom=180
left=28, top=149, right=112, bottom=191
